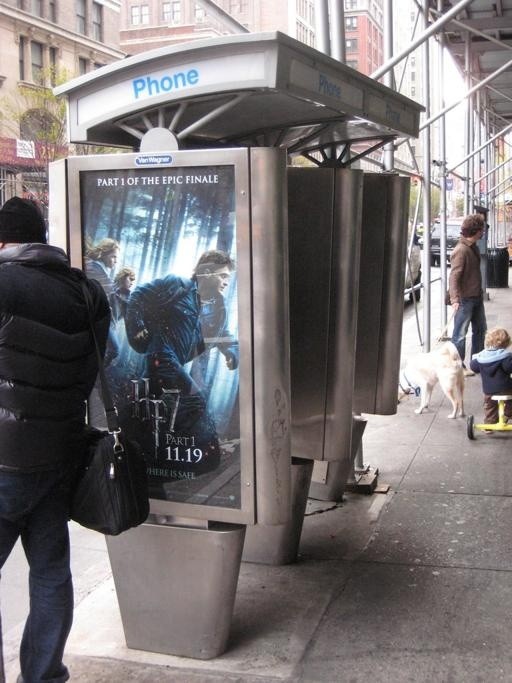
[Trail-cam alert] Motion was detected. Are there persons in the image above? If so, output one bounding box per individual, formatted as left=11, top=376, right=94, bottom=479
left=84, top=235, right=121, bottom=369
left=447, top=214, right=488, bottom=377
left=469, top=328, right=512, bottom=434
left=507, top=232, right=512, bottom=257
left=472, top=206, right=490, bottom=239
left=125, top=248, right=239, bottom=436
left=113, top=267, right=136, bottom=321
left=1, top=197, right=114, bottom=681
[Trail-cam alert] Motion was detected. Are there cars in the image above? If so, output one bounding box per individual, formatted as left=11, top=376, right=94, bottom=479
left=404, top=212, right=467, bottom=303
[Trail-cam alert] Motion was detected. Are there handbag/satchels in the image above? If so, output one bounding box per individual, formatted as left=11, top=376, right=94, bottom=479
left=74, top=279, right=150, bottom=536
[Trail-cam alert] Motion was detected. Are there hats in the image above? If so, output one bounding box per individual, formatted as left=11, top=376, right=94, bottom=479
left=0, top=196, right=47, bottom=244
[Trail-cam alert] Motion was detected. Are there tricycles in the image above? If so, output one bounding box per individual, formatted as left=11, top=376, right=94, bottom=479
left=466, top=391, right=512, bottom=440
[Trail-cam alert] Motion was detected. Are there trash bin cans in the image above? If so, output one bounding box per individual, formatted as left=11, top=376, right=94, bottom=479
left=486, top=247, right=509, bottom=288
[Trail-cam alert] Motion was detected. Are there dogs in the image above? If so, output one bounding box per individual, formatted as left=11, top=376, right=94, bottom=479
left=397, top=340, right=466, bottom=419
